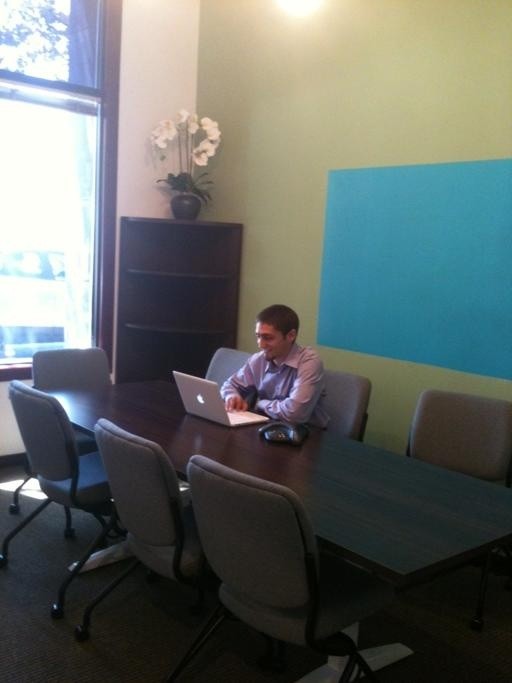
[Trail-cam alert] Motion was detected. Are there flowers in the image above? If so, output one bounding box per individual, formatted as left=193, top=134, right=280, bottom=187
left=148, top=107, right=223, bottom=205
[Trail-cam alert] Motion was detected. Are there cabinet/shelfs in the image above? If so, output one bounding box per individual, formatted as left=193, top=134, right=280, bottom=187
left=114, top=216, right=244, bottom=383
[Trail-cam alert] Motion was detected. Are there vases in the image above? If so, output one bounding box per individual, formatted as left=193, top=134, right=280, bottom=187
left=169, top=192, right=203, bottom=220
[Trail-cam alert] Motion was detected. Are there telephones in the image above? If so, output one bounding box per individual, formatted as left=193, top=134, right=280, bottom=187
left=258, top=421, right=310, bottom=446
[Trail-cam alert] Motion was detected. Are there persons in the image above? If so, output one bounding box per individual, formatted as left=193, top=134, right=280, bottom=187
left=219, top=304, right=332, bottom=430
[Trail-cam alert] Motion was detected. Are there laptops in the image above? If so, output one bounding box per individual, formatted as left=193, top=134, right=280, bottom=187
left=172, top=369, right=270, bottom=428
